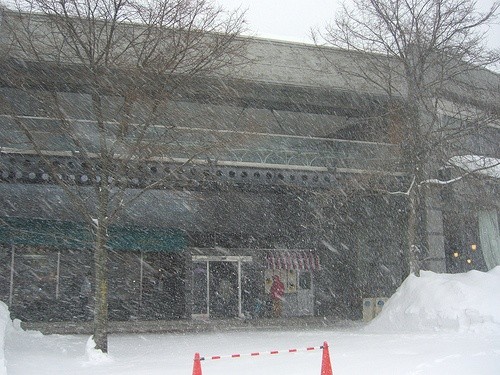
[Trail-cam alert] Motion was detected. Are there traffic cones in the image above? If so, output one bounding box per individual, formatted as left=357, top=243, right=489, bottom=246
left=193, top=353, right=204, bottom=375
left=321, top=342, right=334, bottom=375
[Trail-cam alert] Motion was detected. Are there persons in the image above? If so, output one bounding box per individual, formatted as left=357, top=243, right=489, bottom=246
left=251, top=274, right=284, bottom=314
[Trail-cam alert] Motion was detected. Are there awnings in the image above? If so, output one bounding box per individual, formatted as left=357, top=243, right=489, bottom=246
left=260, top=249, right=321, bottom=273
left=1, top=217, right=187, bottom=253
